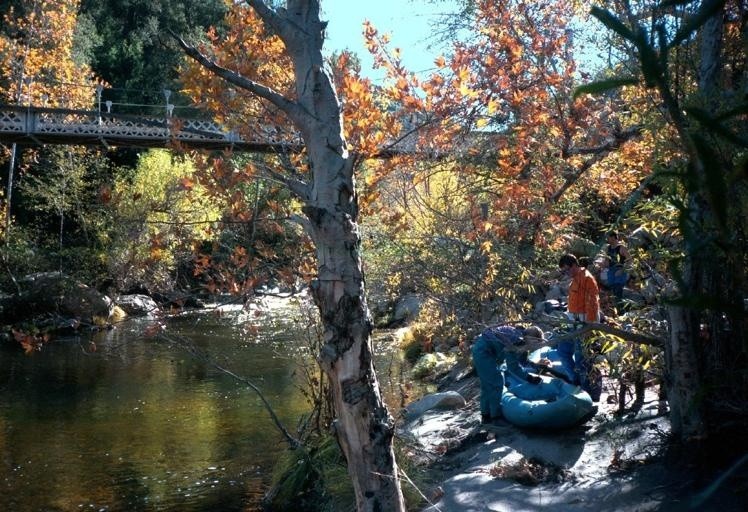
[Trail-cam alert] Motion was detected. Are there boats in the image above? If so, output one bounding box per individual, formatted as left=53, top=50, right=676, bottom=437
left=498, top=344, right=598, bottom=429
left=399, top=390, right=466, bottom=422
left=213, top=302, right=273, bottom=334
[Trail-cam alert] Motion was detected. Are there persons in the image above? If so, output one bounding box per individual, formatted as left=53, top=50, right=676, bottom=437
left=558, top=254, right=602, bottom=403
left=600, top=230, right=634, bottom=316
left=470, top=325, right=552, bottom=427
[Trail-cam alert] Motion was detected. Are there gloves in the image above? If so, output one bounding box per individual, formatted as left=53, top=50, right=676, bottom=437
left=527, top=375, right=542, bottom=386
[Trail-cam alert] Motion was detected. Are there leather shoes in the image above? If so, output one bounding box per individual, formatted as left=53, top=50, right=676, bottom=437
left=481, top=414, right=492, bottom=425
left=491, top=418, right=512, bottom=430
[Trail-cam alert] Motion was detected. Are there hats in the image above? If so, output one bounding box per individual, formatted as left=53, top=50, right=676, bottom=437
left=594, top=258, right=605, bottom=265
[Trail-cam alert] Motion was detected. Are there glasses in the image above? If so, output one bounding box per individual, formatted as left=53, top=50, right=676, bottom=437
left=558, top=267, right=571, bottom=274
